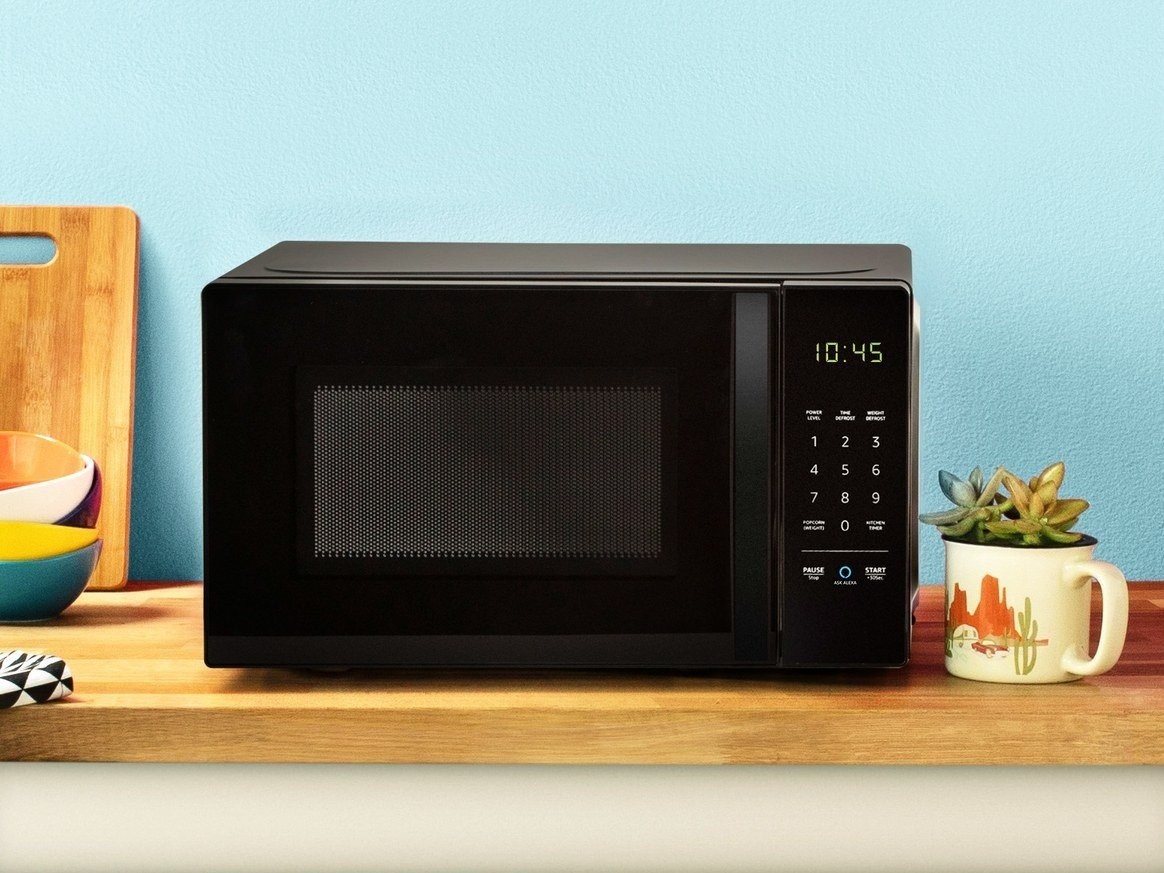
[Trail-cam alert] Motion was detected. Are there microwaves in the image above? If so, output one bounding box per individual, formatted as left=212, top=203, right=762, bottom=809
left=199, top=238, right=919, bottom=684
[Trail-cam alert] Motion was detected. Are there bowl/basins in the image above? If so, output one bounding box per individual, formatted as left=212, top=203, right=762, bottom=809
left=0, top=430, right=103, bottom=624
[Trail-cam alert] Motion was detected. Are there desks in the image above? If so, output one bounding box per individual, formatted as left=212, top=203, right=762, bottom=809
left=0, top=578, right=1163, bottom=873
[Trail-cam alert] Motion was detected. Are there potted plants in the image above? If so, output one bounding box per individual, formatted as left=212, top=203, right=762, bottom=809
left=920, top=464, right=1130, bottom=685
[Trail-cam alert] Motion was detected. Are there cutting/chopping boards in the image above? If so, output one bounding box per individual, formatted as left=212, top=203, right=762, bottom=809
left=1, top=204, right=142, bottom=590
